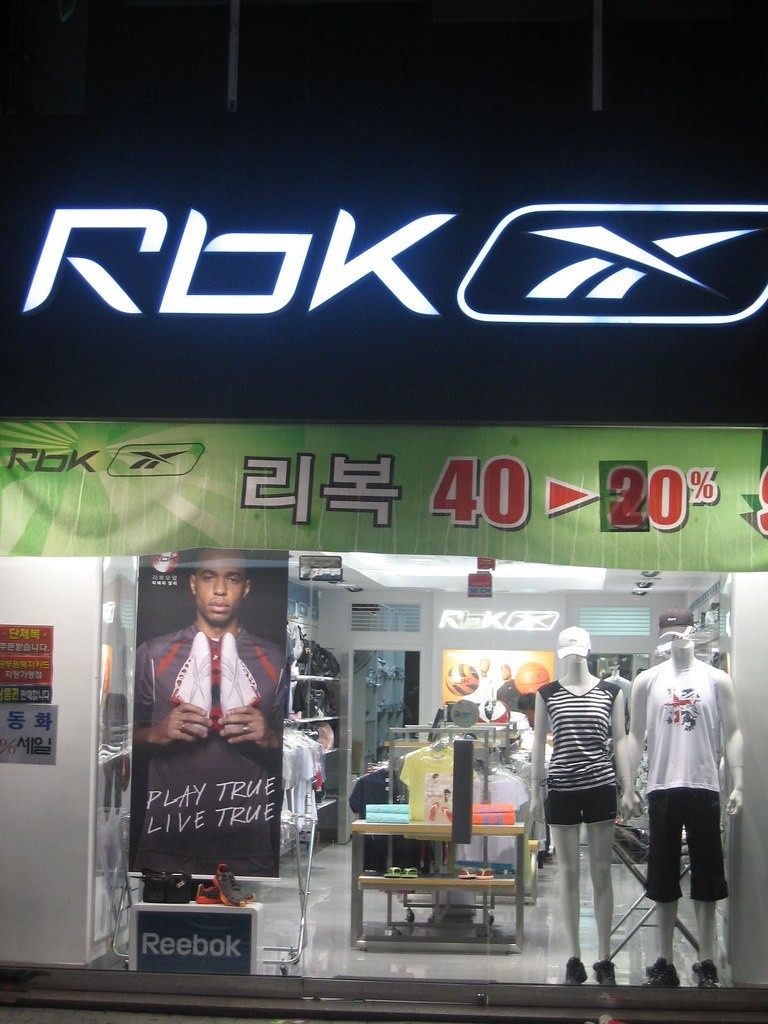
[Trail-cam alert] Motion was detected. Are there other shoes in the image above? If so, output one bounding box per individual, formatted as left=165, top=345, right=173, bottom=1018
left=141, top=872, right=165, bottom=903
left=165, top=871, right=193, bottom=904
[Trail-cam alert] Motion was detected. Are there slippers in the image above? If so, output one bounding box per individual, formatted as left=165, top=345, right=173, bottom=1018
left=402, top=867, right=419, bottom=878
left=384, top=867, right=401, bottom=878
left=458, top=867, right=477, bottom=878
left=477, top=868, right=494, bottom=880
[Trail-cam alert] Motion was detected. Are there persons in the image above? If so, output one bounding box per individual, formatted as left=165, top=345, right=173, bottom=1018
left=464, top=660, right=494, bottom=701
left=496, top=664, right=520, bottom=712
left=529, top=662, right=635, bottom=984
left=603, top=657, right=631, bottom=697
left=101, top=693, right=127, bottom=819
left=132, top=548, right=285, bottom=878
left=629, top=639, right=744, bottom=987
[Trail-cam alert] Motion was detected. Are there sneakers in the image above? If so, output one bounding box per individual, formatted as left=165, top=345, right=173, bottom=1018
left=642, top=958, right=680, bottom=985
left=593, top=957, right=617, bottom=985
left=691, top=958, right=721, bottom=987
left=213, top=863, right=246, bottom=907
left=171, top=631, right=215, bottom=738
left=562, top=957, right=588, bottom=985
left=196, top=884, right=256, bottom=904
left=216, top=632, right=260, bottom=729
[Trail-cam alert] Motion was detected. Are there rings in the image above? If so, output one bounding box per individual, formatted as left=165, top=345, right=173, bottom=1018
left=244, top=723, right=248, bottom=734
left=181, top=723, right=184, bottom=731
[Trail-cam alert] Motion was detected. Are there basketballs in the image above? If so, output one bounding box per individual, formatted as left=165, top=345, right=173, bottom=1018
left=515, top=661, right=550, bottom=694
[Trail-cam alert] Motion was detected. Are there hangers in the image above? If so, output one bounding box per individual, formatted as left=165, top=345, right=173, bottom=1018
left=352, top=736, right=533, bottom=783
left=283, top=719, right=323, bottom=752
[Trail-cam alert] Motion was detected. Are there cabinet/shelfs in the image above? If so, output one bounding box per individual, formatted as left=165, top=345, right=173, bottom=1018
left=352, top=650, right=405, bottom=777
left=288, top=672, right=338, bottom=811
left=350, top=818, right=523, bottom=954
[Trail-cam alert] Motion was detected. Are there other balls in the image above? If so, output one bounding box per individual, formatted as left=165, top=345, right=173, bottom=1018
left=446, top=664, right=481, bottom=696
left=478, top=699, right=510, bottom=723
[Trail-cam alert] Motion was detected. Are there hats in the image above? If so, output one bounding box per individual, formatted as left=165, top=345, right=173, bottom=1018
left=658, top=605, right=696, bottom=638
left=607, top=657, right=621, bottom=669
left=556, top=625, right=592, bottom=659
